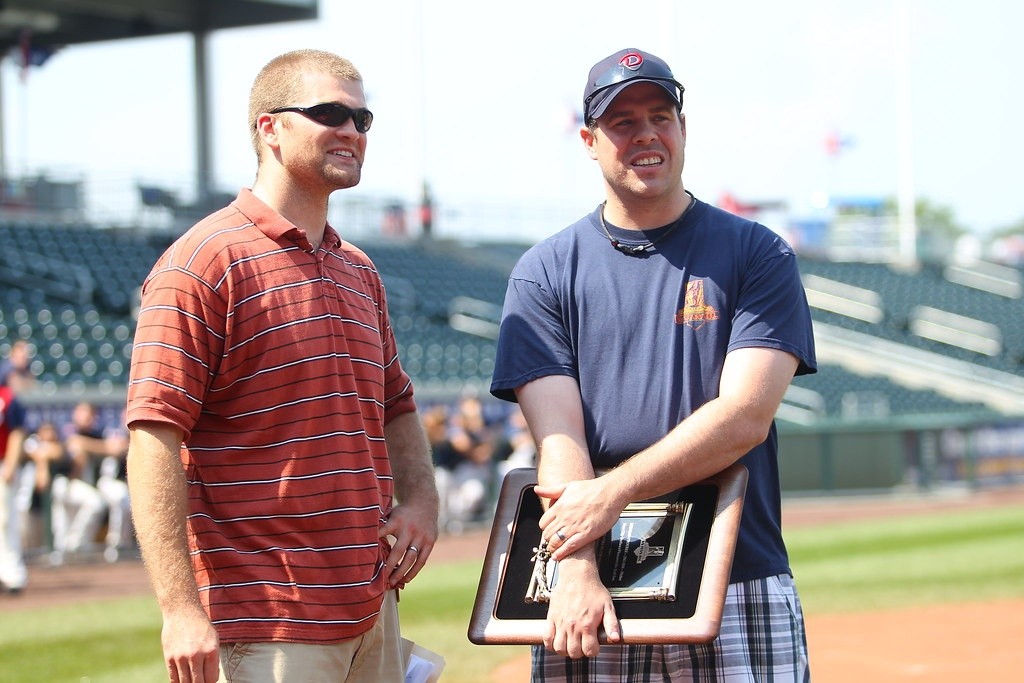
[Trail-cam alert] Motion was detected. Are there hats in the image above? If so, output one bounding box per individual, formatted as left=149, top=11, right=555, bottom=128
left=582, top=48, right=681, bottom=127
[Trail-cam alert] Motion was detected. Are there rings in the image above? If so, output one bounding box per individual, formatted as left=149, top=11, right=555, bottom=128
left=407, top=546, right=418, bottom=552
left=557, top=530, right=566, bottom=540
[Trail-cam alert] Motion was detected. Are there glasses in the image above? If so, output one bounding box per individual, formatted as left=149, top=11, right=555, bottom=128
left=254, top=103, right=374, bottom=134
left=584, top=60, right=685, bottom=126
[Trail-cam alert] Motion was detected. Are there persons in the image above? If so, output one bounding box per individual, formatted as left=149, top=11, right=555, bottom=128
left=0, top=341, right=140, bottom=593
left=127, top=49, right=441, bottom=683
left=490, top=47, right=818, bottom=683
left=418, top=396, right=537, bottom=536
